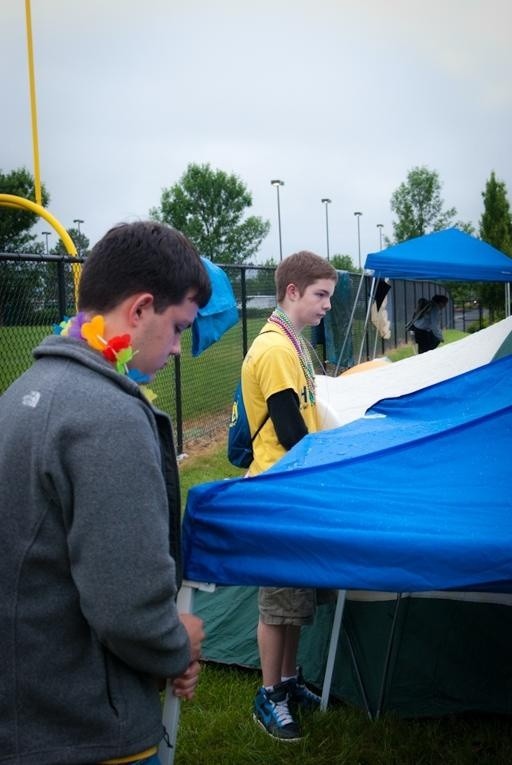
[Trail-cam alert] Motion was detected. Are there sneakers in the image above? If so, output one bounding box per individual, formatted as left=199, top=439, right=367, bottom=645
left=251, top=677, right=311, bottom=743
left=282, top=665, right=347, bottom=712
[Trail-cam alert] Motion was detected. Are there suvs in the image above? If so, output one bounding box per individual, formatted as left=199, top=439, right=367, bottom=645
left=0, top=270, right=75, bottom=316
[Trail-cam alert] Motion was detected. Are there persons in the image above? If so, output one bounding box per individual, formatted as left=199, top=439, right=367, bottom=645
left=240, top=248, right=341, bottom=745
left=407, top=293, right=450, bottom=353
left=2, top=218, right=211, bottom=764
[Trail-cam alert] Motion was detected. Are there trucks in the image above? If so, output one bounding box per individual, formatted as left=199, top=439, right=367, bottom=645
left=234, top=293, right=277, bottom=310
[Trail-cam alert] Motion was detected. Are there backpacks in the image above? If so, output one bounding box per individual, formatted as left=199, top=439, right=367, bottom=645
left=228, top=330, right=310, bottom=468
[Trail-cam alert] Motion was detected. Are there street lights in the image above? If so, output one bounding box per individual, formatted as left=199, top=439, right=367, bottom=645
left=353, top=211, right=364, bottom=273
left=42, top=231, right=52, bottom=256
left=73, top=218, right=85, bottom=256
left=270, top=179, right=286, bottom=263
left=320, top=197, right=332, bottom=261
left=376, top=222, right=385, bottom=250
